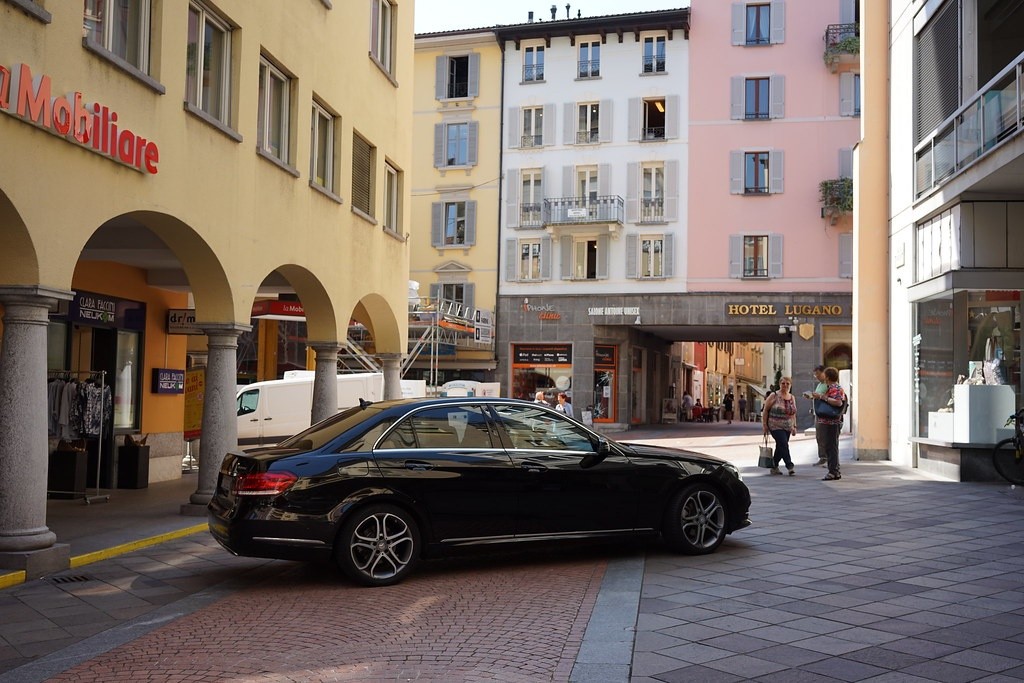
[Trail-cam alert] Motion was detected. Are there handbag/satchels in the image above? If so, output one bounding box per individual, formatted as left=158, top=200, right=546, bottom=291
left=758, top=432, right=775, bottom=468
left=814, top=386, right=845, bottom=418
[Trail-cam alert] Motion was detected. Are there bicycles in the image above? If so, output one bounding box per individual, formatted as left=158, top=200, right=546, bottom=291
left=993, top=408, right=1024, bottom=489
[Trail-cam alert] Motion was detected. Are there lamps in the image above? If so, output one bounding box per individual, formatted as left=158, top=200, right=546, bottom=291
left=634, top=315, right=641, bottom=324
left=792, top=317, right=798, bottom=323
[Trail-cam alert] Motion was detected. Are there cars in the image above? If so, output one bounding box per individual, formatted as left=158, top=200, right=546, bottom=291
left=204, top=392, right=754, bottom=588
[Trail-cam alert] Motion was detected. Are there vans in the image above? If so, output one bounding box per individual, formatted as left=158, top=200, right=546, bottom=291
left=237, top=373, right=385, bottom=452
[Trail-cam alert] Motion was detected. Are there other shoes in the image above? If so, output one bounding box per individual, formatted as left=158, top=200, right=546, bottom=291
left=821, top=470, right=841, bottom=480
left=789, top=468, right=796, bottom=476
left=770, top=468, right=783, bottom=475
left=812, top=458, right=828, bottom=468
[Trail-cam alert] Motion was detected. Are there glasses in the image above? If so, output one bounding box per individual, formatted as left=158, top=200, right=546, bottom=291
left=780, top=382, right=791, bottom=385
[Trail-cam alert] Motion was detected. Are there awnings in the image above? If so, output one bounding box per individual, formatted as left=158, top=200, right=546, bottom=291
left=748, top=384, right=767, bottom=398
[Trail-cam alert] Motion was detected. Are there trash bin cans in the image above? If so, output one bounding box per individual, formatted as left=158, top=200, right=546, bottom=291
left=119, top=446, right=150, bottom=489
left=48, top=450, right=87, bottom=498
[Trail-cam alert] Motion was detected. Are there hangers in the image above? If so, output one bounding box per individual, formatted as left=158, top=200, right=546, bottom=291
left=47, top=367, right=103, bottom=383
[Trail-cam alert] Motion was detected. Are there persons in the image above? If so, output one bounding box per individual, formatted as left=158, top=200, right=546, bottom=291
left=682, top=391, right=693, bottom=422
left=533, top=392, right=550, bottom=407
left=812, top=366, right=847, bottom=481
left=738, top=394, right=747, bottom=421
left=555, top=393, right=575, bottom=419
left=723, top=388, right=735, bottom=424
left=803, top=365, right=828, bottom=467
left=694, top=398, right=703, bottom=422
left=761, top=376, right=798, bottom=476
left=760, top=384, right=777, bottom=411
